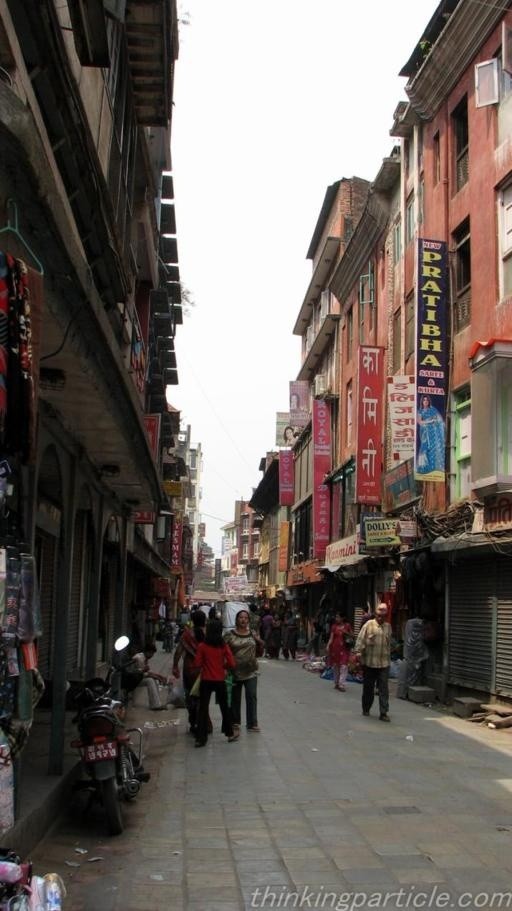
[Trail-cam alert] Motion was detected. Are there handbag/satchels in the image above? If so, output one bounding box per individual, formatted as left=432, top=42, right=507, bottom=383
left=255, top=642, right=265, bottom=657
left=343, top=633, right=356, bottom=648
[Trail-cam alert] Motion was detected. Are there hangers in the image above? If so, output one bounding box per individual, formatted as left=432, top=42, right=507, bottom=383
left=0, top=198, right=45, bottom=277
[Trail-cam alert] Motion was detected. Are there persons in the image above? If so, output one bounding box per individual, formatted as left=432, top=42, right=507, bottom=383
left=129, top=591, right=336, bottom=663
left=121, top=644, right=169, bottom=712
left=325, top=612, right=354, bottom=694
left=221, top=611, right=266, bottom=734
left=354, top=604, right=372, bottom=685
left=185, top=619, right=240, bottom=748
left=291, top=394, right=300, bottom=411
left=416, top=394, right=445, bottom=475
left=283, top=426, right=299, bottom=447
left=354, top=604, right=392, bottom=723
left=172, top=610, right=214, bottom=734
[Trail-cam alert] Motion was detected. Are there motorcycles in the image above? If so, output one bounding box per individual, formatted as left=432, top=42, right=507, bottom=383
left=64, top=632, right=154, bottom=839
left=159, top=620, right=175, bottom=653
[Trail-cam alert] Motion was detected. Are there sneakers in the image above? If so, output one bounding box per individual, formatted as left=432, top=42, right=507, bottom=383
left=361, top=707, right=370, bottom=716
left=335, top=684, right=346, bottom=692
left=378, top=714, right=391, bottom=722
left=150, top=705, right=168, bottom=711
left=189, top=722, right=262, bottom=749
